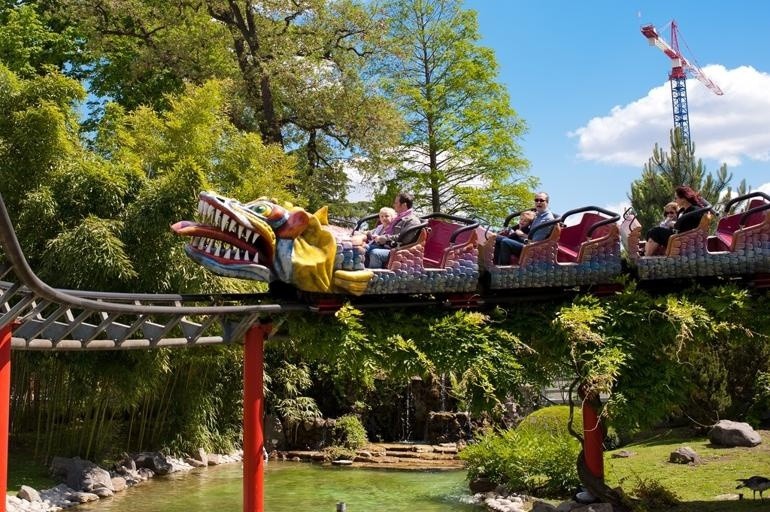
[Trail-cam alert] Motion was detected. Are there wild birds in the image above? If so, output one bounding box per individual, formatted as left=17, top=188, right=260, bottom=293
left=736, top=475, right=770, bottom=501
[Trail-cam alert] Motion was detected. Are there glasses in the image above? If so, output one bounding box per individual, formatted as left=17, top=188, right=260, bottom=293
left=535, top=198, right=547, bottom=202
left=666, top=211, right=675, bottom=215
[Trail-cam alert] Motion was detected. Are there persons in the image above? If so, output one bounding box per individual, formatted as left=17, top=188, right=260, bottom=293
left=659, top=201, right=680, bottom=228
left=363, top=207, right=396, bottom=244
left=639, top=186, right=703, bottom=257
left=363, top=191, right=422, bottom=268
left=497, top=209, right=536, bottom=236
left=491, top=191, right=556, bottom=266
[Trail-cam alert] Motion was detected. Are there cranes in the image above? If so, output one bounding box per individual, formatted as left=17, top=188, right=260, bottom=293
left=639, top=17, right=724, bottom=177
